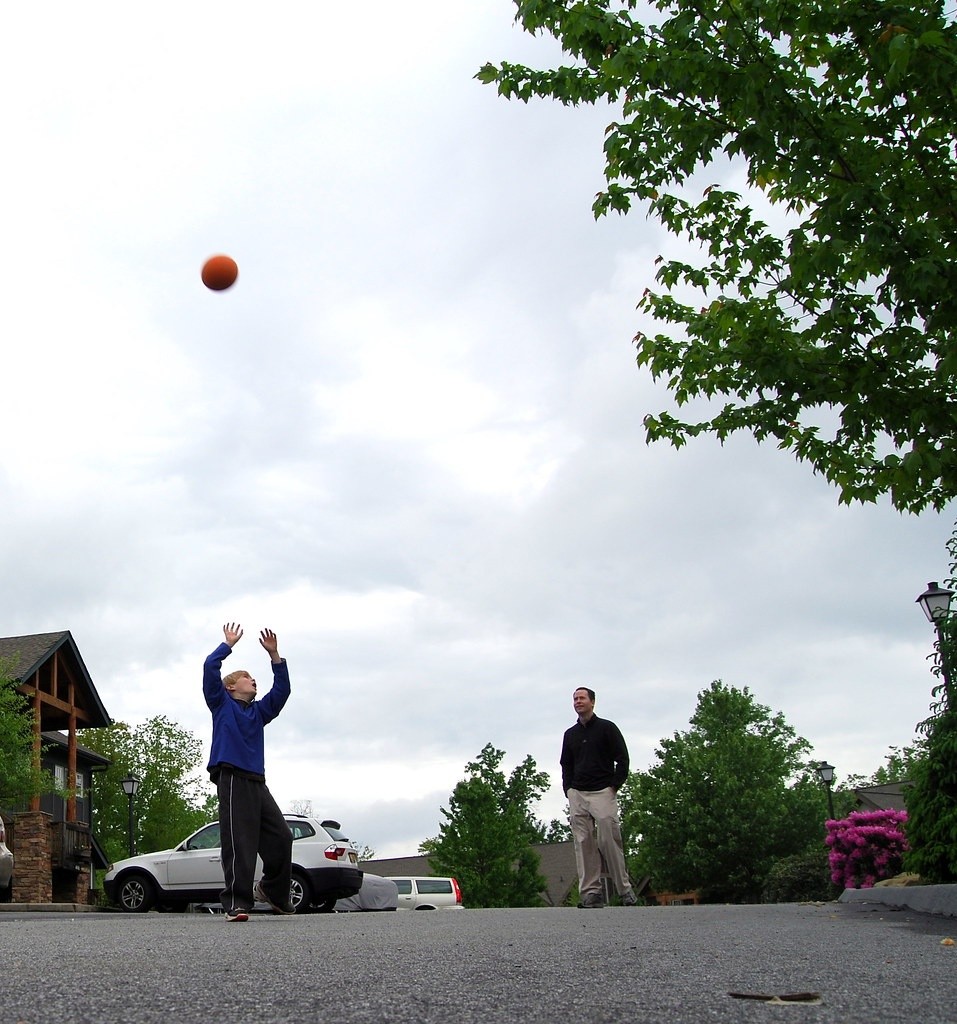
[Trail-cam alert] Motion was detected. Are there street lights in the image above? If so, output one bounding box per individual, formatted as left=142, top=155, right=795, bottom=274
left=119, top=773, right=140, bottom=857
left=916, top=581, right=957, bottom=730
left=816, top=760, right=837, bottom=821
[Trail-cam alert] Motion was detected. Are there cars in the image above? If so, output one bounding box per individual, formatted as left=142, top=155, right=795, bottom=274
left=381, top=877, right=465, bottom=911
left=0, top=815, right=14, bottom=903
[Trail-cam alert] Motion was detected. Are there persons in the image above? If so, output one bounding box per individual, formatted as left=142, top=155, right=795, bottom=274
left=204, top=622, right=296, bottom=923
left=560, top=687, right=639, bottom=909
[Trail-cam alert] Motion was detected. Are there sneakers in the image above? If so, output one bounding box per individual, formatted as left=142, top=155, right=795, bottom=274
left=224, top=908, right=248, bottom=922
left=623, top=888, right=637, bottom=905
left=577, top=894, right=604, bottom=909
left=256, top=881, right=296, bottom=914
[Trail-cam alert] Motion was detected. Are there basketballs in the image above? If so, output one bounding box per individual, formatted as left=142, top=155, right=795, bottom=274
left=201, top=256, right=238, bottom=290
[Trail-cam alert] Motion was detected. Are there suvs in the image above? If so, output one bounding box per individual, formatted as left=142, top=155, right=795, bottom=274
left=103, top=813, right=365, bottom=915
